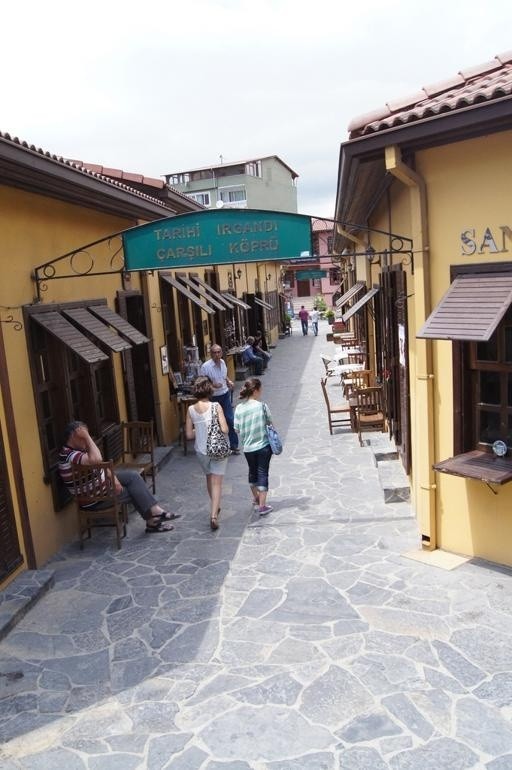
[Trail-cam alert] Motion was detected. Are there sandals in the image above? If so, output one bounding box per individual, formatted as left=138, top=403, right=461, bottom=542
left=145, top=511, right=181, bottom=532
left=211, top=508, right=220, bottom=529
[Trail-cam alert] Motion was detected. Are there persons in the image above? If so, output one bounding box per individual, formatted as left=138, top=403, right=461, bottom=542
left=298, top=305, right=311, bottom=337
left=230, top=376, right=277, bottom=518
left=253, top=330, right=273, bottom=369
left=183, top=375, right=232, bottom=530
left=242, top=335, right=264, bottom=375
left=198, top=342, right=241, bottom=455
left=310, top=305, right=320, bottom=337
left=56, top=419, right=183, bottom=535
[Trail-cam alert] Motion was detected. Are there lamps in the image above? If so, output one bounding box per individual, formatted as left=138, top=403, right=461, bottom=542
left=366, top=245, right=381, bottom=266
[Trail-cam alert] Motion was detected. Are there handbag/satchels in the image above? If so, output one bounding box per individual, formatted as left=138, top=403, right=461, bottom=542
left=207, top=423, right=232, bottom=459
left=266, top=424, right=282, bottom=455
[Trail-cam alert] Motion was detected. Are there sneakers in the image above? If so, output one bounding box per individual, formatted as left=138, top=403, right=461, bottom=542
left=252, top=495, right=273, bottom=514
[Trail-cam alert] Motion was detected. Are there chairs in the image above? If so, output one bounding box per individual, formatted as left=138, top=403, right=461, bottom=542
left=70, top=398, right=199, bottom=551
left=320, top=339, right=385, bottom=447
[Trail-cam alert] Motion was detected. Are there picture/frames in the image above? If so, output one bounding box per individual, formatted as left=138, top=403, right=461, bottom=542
left=160, top=344, right=170, bottom=375
